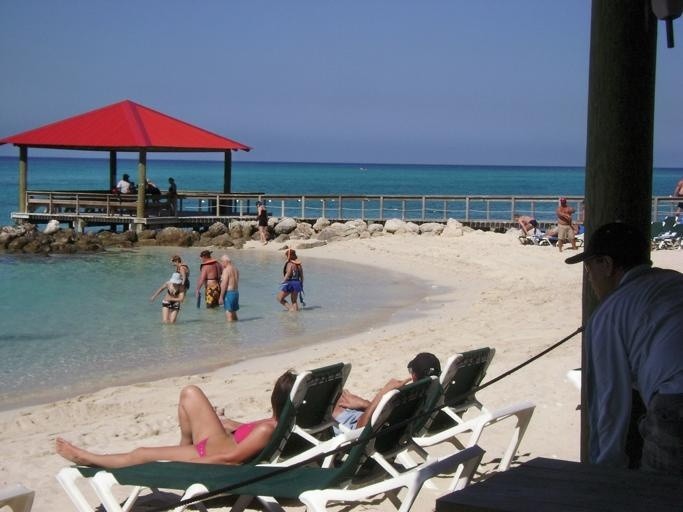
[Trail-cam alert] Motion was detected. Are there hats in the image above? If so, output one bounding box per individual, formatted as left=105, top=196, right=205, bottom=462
left=407, top=353, right=440, bottom=374
left=565, top=222, right=643, bottom=264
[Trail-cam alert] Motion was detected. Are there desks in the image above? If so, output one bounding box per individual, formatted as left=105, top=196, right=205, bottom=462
left=436, top=458, right=683, bottom=512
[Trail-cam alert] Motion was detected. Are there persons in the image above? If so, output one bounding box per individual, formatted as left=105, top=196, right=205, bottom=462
left=150, top=271, right=186, bottom=325
left=513, top=214, right=538, bottom=245
left=195, top=249, right=223, bottom=309
left=564, top=219, right=682, bottom=477
left=556, top=197, right=578, bottom=252
left=255, top=201, right=269, bottom=246
left=111, top=171, right=180, bottom=217
left=218, top=255, right=240, bottom=323
left=56, top=370, right=298, bottom=472
left=171, top=254, right=190, bottom=291
left=330, top=352, right=443, bottom=431
left=277, top=249, right=304, bottom=313
left=541, top=221, right=580, bottom=237
left=673, top=179, right=683, bottom=223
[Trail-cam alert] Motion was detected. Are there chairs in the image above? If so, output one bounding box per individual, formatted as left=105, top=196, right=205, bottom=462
left=518, top=224, right=584, bottom=248
left=650, top=214, right=683, bottom=251
